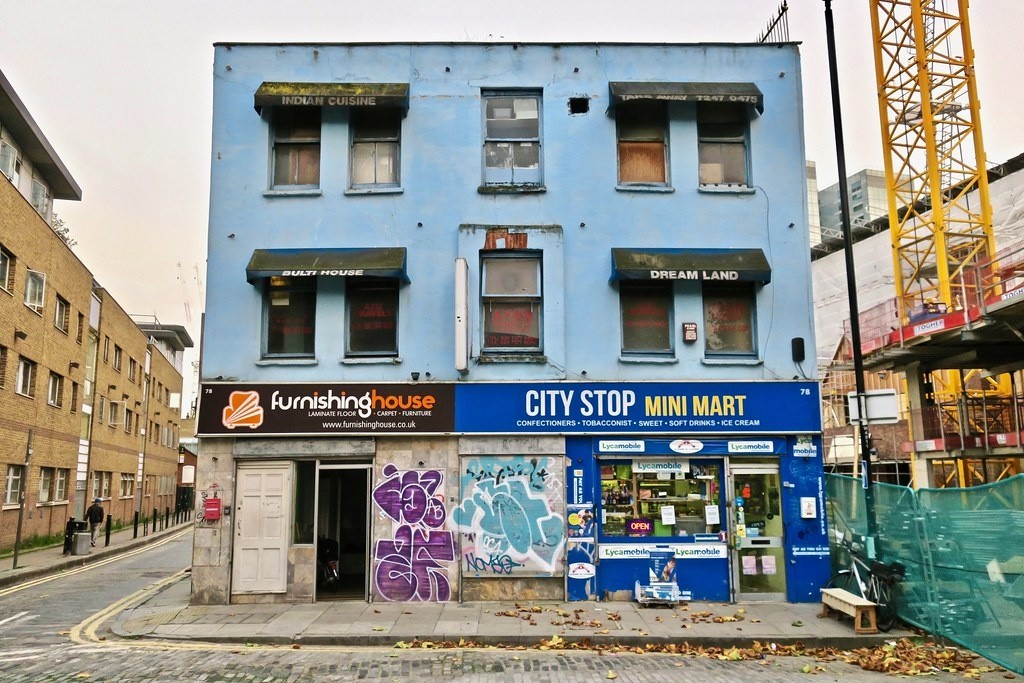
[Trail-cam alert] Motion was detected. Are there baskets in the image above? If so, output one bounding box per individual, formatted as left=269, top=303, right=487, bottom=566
left=829, top=542, right=857, bottom=565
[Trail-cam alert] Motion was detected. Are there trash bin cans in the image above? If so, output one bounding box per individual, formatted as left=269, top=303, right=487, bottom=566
left=64, top=521, right=88, bottom=554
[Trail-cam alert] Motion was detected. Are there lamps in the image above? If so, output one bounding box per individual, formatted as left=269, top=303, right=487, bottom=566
left=154, top=412, right=160, bottom=417
left=173, top=423, right=177, bottom=427
left=134, top=400, right=142, bottom=408
left=69, top=360, right=80, bottom=372
left=123, top=393, right=129, bottom=400
left=168, top=420, right=173, bottom=424
left=107, top=384, right=118, bottom=391
left=13, top=330, right=27, bottom=341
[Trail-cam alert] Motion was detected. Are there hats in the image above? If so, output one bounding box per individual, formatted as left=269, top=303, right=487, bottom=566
left=95, top=498, right=101, bottom=503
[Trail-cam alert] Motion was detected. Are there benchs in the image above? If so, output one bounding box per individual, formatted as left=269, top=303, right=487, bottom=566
left=820, top=587, right=878, bottom=635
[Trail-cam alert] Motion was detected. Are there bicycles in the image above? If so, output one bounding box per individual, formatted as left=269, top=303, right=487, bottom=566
left=824, top=539, right=905, bottom=632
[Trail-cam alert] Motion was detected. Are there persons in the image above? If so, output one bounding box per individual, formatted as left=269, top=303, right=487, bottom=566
left=82, top=498, right=104, bottom=547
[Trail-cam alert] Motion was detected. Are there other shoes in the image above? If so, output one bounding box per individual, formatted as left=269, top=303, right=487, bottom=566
left=91, top=540, right=96, bottom=548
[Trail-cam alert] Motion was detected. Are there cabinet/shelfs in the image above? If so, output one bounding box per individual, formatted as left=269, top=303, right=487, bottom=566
left=601, top=472, right=714, bottom=535
